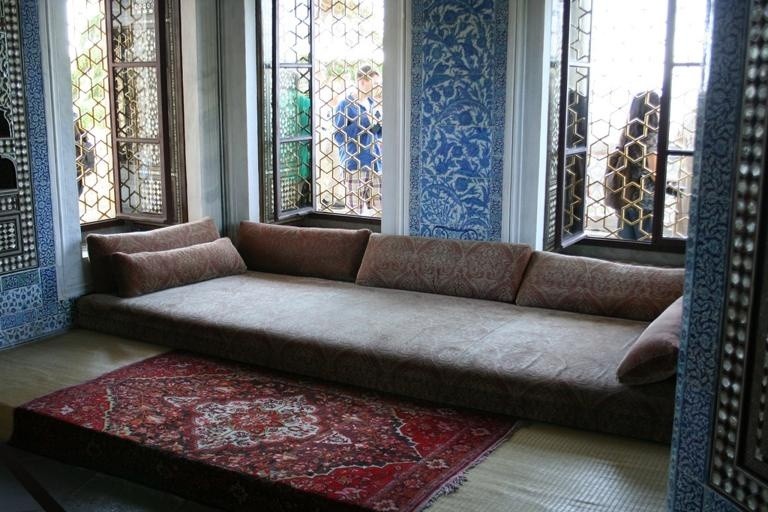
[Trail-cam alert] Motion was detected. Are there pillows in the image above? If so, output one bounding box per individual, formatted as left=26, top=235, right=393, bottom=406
left=113, top=237, right=248, bottom=298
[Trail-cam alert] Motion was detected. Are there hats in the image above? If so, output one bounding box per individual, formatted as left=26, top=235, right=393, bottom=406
left=358, top=66, right=379, bottom=77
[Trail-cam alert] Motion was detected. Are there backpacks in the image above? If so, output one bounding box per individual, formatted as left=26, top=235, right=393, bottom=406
left=604, top=146, right=642, bottom=210
left=76, top=142, right=94, bottom=176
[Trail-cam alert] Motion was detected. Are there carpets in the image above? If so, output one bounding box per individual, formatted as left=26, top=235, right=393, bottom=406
left=7, top=348, right=527, bottom=512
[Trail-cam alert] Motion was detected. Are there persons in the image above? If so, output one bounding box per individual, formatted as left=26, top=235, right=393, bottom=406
left=603, top=91, right=685, bottom=243
left=279, top=69, right=312, bottom=204
left=73, top=105, right=95, bottom=197
left=335, top=65, right=383, bottom=213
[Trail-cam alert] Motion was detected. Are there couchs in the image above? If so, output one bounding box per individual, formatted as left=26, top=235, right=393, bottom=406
left=76, top=218, right=685, bottom=446
